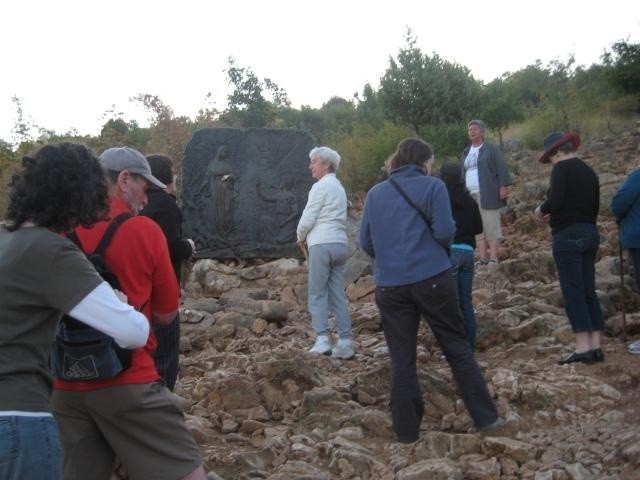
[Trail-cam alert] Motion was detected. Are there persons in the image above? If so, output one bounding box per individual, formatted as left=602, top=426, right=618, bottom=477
left=360, top=138, right=502, bottom=443
left=439, top=161, right=482, bottom=360
left=0, top=140, right=149, bottom=480
left=295, top=144, right=355, bottom=361
left=141, top=155, right=197, bottom=390
left=535, top=132, right=605, bottom=365
left=50, top=144, right=207, bottom=479
left=254, top=174, right=297, bottom=244
left=609, top=149, right=639, bottom=355
left=462, top=120, right=512, bottom=265
left=204, top=145, right=236, bottom=248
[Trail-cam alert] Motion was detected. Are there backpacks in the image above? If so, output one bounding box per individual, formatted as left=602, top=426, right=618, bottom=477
left=49, top=211, right=135, bottom=383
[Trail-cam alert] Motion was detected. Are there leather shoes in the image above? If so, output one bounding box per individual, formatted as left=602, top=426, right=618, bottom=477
left=595, top=348, right=603, bottom=361
left=557, top=349, right=595, bottom=366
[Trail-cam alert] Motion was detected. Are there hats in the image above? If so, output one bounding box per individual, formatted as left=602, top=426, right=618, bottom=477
left=98, top=145, right=168, bottom=190
left=538, top=131, right=581, bottom=165
left=147, top=154, right=174, bottom=177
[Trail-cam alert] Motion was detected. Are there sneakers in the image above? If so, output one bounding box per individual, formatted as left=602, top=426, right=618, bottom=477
left=479, top=416, right=517, bottom=430
left=488, top=259, right=498, bottom=267
left=478, top=258, right=487, bottom=268
left=628, top=340, right=640, bottom=354
left=333, top=342, right=355, bottom=360
left=310, top=337, right=333, bottom=356
